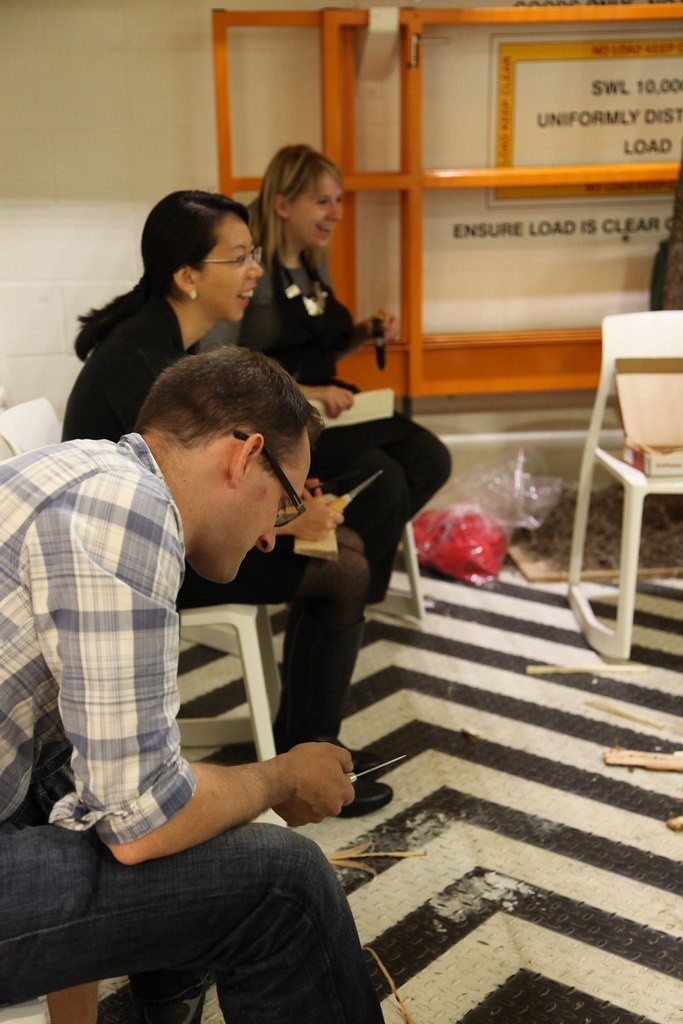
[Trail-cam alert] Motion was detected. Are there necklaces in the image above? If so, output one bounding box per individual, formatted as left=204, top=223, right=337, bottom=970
left=278, top=260, right=327, bottom=317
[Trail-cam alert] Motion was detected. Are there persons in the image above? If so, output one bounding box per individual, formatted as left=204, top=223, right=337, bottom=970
left=61, top=189, right=393, bottom=820
left=0, top=345, right=384, bottom=1024
left=198, top=143, right=452, bottom=605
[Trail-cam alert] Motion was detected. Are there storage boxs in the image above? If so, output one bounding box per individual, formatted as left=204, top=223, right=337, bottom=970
left=613, top=358, right=683, bottom=480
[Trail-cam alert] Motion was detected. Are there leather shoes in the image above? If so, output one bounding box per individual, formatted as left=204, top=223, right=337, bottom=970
left=335, top=740, right=387, bottom=776
left=338, top=778, right=393, bottom=818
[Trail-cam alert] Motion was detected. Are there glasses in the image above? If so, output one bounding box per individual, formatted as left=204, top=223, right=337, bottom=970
left=232, top=429, right=306, bottom=528
left=201, top=244, right=263, bottom=269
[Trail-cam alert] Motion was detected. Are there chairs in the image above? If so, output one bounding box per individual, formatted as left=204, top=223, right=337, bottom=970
left=569, top=308, right=683, bottom=662
left=0, top=399, right=428, bottom=1023
